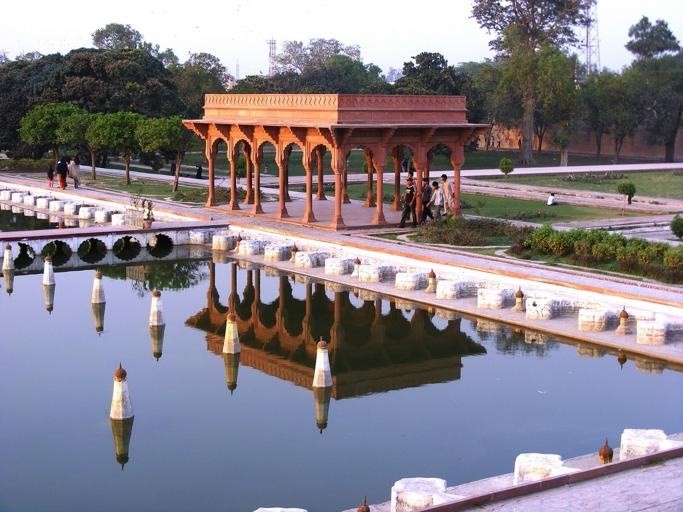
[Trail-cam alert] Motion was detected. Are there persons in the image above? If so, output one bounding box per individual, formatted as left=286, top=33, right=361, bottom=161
left=420, top=177, right=434, bottom=223
left=45, top=163, right=54, bottom=188
left=398, top=176, right=417, bottom=228
left=545, top=191, right=558, bottom=206
left=475, top=135, right=480, bottom=148
left=67, top=156, right=81, bottom=188
left=405, top=170, right=416, bottom=192
left=490, top=137, right=494, bottom=148
left=495, top=138, right=500, bottom=148
left=425, top=180, right=444, bottom=219
left=516, top=138, right=520, bottom=149
left=194, top=164, right=203, bottom=178
left=54, top=158, right=69, bottom=190
left=439, top=174, right=454, bottom=217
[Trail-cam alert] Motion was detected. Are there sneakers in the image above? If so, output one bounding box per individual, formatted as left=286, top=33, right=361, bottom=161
left=394, top=222, right=417, bottom=228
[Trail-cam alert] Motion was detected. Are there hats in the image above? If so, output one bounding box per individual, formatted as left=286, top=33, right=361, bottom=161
left=405, top=178, right=414, bottom=182
